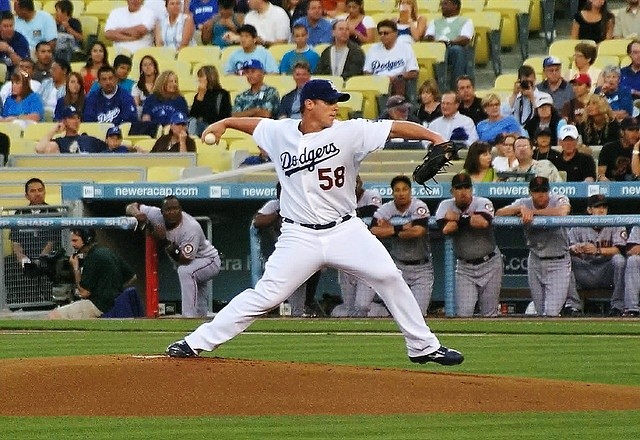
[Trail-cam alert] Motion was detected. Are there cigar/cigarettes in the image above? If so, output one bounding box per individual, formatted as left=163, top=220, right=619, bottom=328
left=140, top=223, right=147, bottom=231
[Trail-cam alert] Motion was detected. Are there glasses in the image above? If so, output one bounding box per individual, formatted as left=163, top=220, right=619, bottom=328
left=379, top=31, right=392, bottom=35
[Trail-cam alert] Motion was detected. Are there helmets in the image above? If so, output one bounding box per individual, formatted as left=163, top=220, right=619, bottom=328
left=169, top=109, right=191, bottom=125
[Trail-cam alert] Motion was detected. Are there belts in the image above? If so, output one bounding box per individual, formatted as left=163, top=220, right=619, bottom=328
left=457, top=252, right=494, bottom=264
left=402, top=258, right=428, bottom=265
left=285, top=214, right=352, bottom=230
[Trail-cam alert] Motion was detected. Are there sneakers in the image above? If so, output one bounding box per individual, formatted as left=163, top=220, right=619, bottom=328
left=408, top=346, right=465, bottom=367
left=164, top=340, right=197, bottom=360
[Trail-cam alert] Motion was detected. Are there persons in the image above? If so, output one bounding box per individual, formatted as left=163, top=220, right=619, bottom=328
left=562, top=194, right=628, bottom=319
left=327, top=175, right=382, bottom=318
left=190, top=0, right=369, bottom=129
left=369, top=176, right=435, bottom=319
left=571, top=0, right=614, bottom=42
left=575, top=93, right=622, bottom=146
left=564, top=74, right=596, bottom=125
left=613, top=1, right=640, bottom=40
left=597, top=117, right=639, bottom=181
left=127, top=194, right=221, bottom=320
left=594, top=65, right=634, bottom=119
left=50, top=226, right=137, bottom=318
left=623, top=213, right=640, bottom=319
left=460, top=124, right=596, bottom=184
left=365, top=0, right=475, bottom=90
left=380, top=57, right=573, bottom=144
left=569, top=42, right=605, bottom=91
left=621, top=40, right=640, bottom=97
left=435, top=172, right=504, bottom=317
left=0, top=0, right=198, bottom=155
left=496, top=176, right=572, bottom=317
left=254, top=177, right=321, bottom=317
left=167, top=79, right=464, bottom=366
left=9, top=177, right=62, bottom=278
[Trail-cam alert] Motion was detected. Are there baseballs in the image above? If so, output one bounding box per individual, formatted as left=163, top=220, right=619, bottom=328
left=205, top=133, right=216, bottom=145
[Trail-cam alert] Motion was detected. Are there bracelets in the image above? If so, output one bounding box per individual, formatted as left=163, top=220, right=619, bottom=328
left=597, top=248, right=601, bottom=255
left=390, top=234, right=399, bottom=238
left=394, top=224, right=404, bottom=232
left=632, top=149, right=639, bottom=155
left=458, top=212, right=471, bottom=225
left=72, top=267, right=79, bottom=272
left=167, top=242, right=181, bottom=261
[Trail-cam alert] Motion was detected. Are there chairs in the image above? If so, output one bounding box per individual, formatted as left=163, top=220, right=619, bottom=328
left=475, top=40, right=637, bottom=104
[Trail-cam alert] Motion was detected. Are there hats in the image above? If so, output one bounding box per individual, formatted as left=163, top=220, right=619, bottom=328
left=106, top=127, right=122, bottom=138
left=585, top=195, right=610, bottom=208
left=535, top=93, right=554, bottom=108
left=299, top=79, right=350, bottom=103
left=529, top=176, right=550, bottom=193
left=558, top=124, right=579, bottom=142
left=569, top=72, right=591, bottom=85
left=452, top=173, right=474, bottom=188
left=541, top=56, right=562, bottom=66
left=241, top=59, right=265, bottom=72
left=534, top=127, right=552, bottom=138
left=385, top=95, right=414, bottom=110
left=62, top=106, right=80, bottom=117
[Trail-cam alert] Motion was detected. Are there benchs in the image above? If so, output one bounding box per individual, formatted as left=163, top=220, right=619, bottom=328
left=1, top=149, right=249, bottom=258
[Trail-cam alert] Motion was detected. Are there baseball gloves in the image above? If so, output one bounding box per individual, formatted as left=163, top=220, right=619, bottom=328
left=412, top=142, right=465, bottom=196
